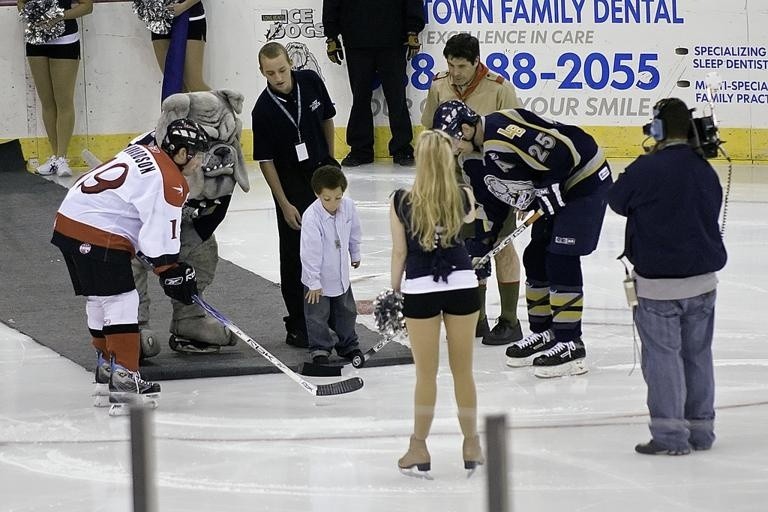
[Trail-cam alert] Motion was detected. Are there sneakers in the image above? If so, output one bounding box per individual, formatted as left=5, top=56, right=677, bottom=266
left=96, top=358, right=160, bottom=393
left=635, top=440, right=690, bottom=455
left=506, top=328, right=556, bottom=357
left=35, top=154, right=71, bottom=177
left=393, top=152, right=414, bottom=166
left=314, top=356, right=329, bottom=364
left=476, top=315, right=523, bottom=345
left=534, top=340, right=585, bottom=365
left=341, top=153, right=374, bottom=167
left=341, top=349, right=363, bottom=361
left=286, top=333, right=308, bottom=348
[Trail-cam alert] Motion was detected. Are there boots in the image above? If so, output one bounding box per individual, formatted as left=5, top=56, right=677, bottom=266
left=398, top=435, right=430, bottom=471
left=462, top=435, right=485, bottom=468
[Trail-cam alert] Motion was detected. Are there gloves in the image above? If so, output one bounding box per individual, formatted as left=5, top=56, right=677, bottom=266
left=327, top=37, right=343, bottom=65
left=407, top=35, right=420, bottom=61
left=535, top=183, right=567, bottom=216
left=160, top=262, right=198, bottom=305
left=466, top=238, right=491, bottom=280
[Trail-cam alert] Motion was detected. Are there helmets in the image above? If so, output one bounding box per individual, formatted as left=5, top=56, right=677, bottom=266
left=435, top=103, right=479, bottom=140
left=163, top=118, right=210, bottom=166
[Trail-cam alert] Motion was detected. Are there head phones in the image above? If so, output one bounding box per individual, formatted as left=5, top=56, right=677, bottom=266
left=650, top=99, right=666, bottom=141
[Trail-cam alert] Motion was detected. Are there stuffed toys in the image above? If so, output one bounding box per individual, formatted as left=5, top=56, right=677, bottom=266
left=127, top=90, right=252, bottom=358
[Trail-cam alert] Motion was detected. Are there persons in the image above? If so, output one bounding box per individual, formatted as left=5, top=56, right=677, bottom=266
left=251, top=42, right=340, bottom=346
left=50, top=119, right=210, bottom=403
left=422, top=33, right=523, bottom=346
left=134, top=0, right=213, bottom=91
left=299, top=166, right=362, bottom=364
left=429, top=99, right=615, bottom=366
left=17, top=0, right=94, bottom=177
left=322, top=0, right=426, bottom=167
left=609, top=98, right=727, bottom=454
left=389, top=129, right=486, bottom=471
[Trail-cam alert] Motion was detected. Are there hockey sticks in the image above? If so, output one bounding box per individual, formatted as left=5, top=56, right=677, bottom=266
left=297, top=207, right=545, bottom=377
left=134, top=250, right=363, bottom=396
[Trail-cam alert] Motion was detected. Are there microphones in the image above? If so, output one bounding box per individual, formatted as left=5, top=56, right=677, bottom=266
left=642, top=135, right=654, bottom=152
